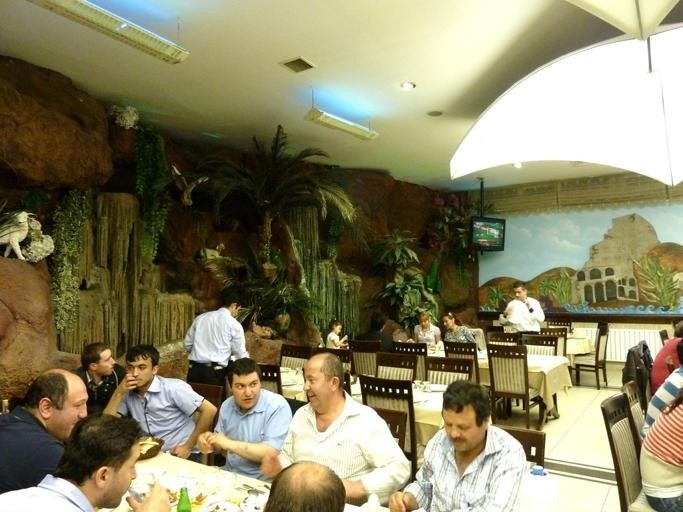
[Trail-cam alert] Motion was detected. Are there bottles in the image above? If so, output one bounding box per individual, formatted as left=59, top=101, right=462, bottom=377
left=177, top=487, right=193, bottom=512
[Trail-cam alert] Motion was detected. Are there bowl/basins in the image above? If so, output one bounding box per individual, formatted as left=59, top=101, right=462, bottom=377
left=132, top=439, right=165, bottom=460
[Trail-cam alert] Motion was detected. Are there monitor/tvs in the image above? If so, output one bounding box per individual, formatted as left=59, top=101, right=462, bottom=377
left=468, top=216, right=505, bottom=251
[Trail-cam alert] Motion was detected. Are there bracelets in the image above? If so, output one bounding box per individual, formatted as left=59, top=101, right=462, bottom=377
left=527, top=307, right=533, bottom=313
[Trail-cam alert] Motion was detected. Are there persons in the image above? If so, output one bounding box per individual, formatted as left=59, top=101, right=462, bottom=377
left=263, top=460, right=346, bottom=511
left=410, top=309, right=440, bottom=347
left=183, top=286, right=251, bottom=387
left=389, top=380, right=529, bottom=512
left=258, top=350, right=412, bottom=505
left=650, top=320, right=682, bottom=390
left=98, top=344, right=219, bottom=464
left=358, top=311, right=394, bottom=350
left=0, top=414, right=172, bottom=511
left=199, top=356, right=292, bottom=483
left=638, top=392, right=683, bottom=512
left=497, top=279, right=546, bottom=340
left=438, top=312, right=482, bottom=348
left=72, top=342, right=126, bottom=414
left=0, top=370, right=89, bottom=493
left=323, top=319, right=349, bottom=350
left=638, top=339, right=683, bottom=437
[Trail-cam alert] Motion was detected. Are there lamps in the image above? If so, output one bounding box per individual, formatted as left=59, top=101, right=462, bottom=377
left=25, top=0, right=191, bottom=65
left=450, top=23, right=683, bottom=188
left=304, top=91, right=378, bottom=141
left=566, top=0, right=680, bottom=41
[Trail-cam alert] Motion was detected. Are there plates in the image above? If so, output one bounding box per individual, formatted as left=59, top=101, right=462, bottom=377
left=241, top=492, right=287, bottom=512
left=400, top=390, right=430, bottom=403
left=204, top=501, right=242, bottom=512
left=427, top=383, right=448, bottom=392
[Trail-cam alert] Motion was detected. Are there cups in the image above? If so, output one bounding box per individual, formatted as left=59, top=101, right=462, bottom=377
left=422, top=381, right=431, bottom=392
left=127, top=474, right=157, bottom=504
left=413, top=380, right=422, bottom=390
